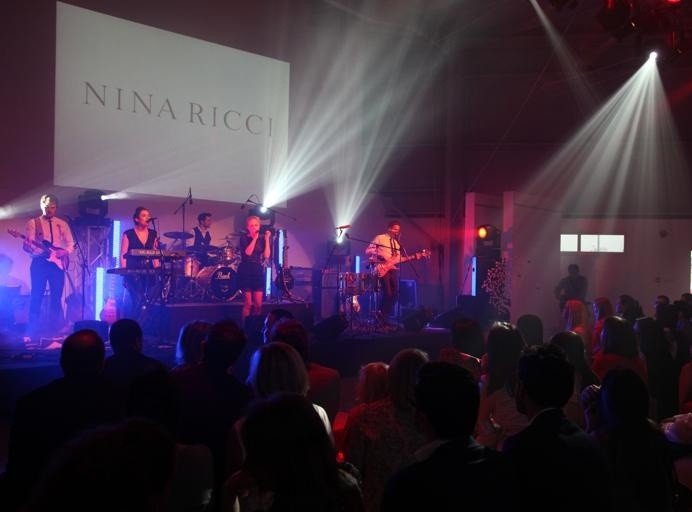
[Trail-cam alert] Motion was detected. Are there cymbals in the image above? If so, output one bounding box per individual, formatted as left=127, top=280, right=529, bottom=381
left=163, top=232, right=195, bottom=239
left=186, top=244, right=217, bottom=253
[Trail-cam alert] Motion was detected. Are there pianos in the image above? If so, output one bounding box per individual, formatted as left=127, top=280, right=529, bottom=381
left=106, top=267, right=186, bottom=276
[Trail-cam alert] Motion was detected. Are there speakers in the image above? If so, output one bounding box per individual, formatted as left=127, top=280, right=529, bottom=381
left=403, top=305, right=433, bottom=330
left=70, top=319, right=110, bottom=344
left=475, top=247, right=501, bottom=313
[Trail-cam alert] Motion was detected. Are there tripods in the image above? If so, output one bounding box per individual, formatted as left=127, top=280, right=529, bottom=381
left=268, top=269, right=306, bottom=303
left=349, top=294, right=387, bottom=339
left=175, top=268, right=207, bottom=302
left=375, top=295, right=398, bottom=334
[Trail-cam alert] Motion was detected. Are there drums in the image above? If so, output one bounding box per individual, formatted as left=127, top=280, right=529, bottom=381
left=215, top=246, right=237, bottom=264
left=195, top=264, right=240, bottom=302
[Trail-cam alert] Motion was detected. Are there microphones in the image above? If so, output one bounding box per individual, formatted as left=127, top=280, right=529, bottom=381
left=188, top=186, right=193, bottom=204
left=331, top=225, right=354, bottom=230
left=240, top=194, right=254, bottom=210
left=345, top=232, right=352, bottom=241
left=147, top=216, right=158, bottom=221
left=61, top=211, right=73, bottom=223
left=253, top=227, right=261, bottom=235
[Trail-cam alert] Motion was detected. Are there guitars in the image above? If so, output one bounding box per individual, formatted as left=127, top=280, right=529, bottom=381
left=7, top=227, right=69, bottom=272
left=375, top=248, right=433, bottom=278
left=274, top=229, right=294, bottom=290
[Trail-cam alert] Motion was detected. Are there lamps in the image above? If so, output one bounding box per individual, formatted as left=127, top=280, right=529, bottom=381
left=248, top=203, right=277, bottom=229
left=75, top=189, right=112, bottom=221
left=477, top=221, right=501, bottom=241
left=325, top=234, right=352, bottom=257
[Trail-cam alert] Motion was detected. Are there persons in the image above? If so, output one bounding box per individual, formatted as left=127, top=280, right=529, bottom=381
left=23, top=191, right=75, bottom=347
left=120, top=207, right=171, bottom=321
left=238, top=215, right=272, bottom=328
left=187, top=211, right=224, bottom=304
left=365, top=220, right=431, bottom=323
left=551, top=264, right=587, bottom=300
